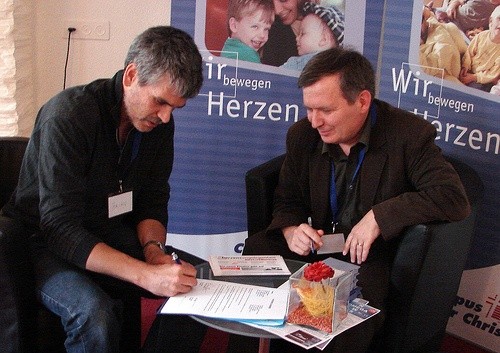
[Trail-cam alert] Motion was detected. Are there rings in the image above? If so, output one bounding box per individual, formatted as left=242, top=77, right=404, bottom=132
left=358, top=243, right=362, bottom=246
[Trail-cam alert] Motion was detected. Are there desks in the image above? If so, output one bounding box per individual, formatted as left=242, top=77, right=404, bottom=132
left=184, top=258, right=374, bottom=353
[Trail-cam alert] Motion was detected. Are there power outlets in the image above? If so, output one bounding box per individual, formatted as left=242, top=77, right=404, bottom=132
left=63, top=20, right=110, bottom=40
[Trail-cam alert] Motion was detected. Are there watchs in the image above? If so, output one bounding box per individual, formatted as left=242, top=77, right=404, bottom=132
left=141, top=240, right=167, bottom=254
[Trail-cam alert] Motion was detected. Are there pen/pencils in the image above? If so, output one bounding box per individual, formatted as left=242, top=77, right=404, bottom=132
left=308, top=217, right=314, bottom=253
left=171, top=252, right=182, bottom=264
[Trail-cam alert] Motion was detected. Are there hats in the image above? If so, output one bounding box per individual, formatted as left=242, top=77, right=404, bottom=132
left=301, top=1, right=345, bottom=44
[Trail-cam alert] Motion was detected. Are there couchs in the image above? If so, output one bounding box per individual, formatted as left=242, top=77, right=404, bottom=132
left=245, top=146, right=485, bottom=353
left=0, top=136, right=208, bottom=353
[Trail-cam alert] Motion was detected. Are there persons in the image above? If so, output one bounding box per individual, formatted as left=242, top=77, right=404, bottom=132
left=0, top=27, right=216, bottom=353
left=262, top=0, right=324, bottom=70
left=421, top=0, right=500, bottom=92
left=219, top=0, right=276, bottom=67
left=279, top=4, right=345, bottom=74
left=223, top=58, right=470, bottom=353
